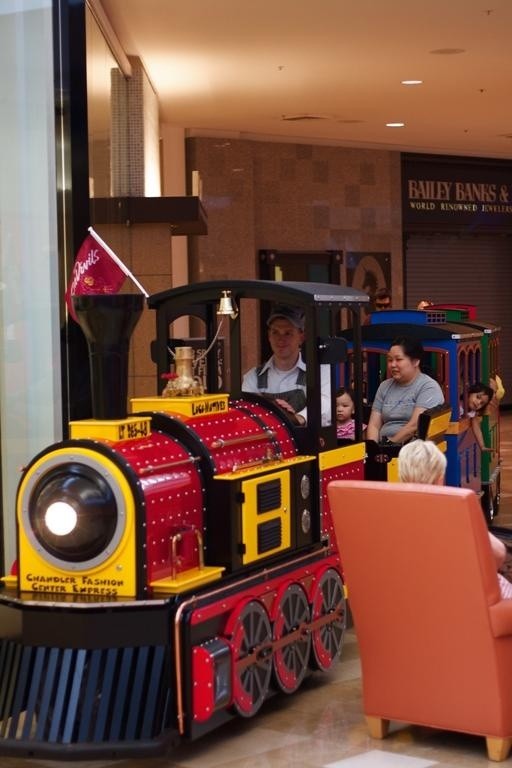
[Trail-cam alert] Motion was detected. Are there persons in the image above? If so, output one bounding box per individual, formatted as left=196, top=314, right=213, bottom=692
left=457, top=380, right=500, bottom=454
left=363, top=336, right=446, bottom=482
left=488, top=376, right=499, bottom=398
left=236, top=299, right=333, bottom=427
left=392, top=438, right=512, bottom=600
left=331, top=386, right=368, bottom=441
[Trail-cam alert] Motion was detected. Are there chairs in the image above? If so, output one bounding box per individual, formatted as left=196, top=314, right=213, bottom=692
left=327, top=483, right=512, bottom=762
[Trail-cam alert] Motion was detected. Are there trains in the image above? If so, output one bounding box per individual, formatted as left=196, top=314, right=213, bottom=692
left=0, top=278, right=506, bottom=762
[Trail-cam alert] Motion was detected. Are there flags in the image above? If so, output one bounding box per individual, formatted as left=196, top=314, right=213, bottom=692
left=62, top=235, right=125, bottom=328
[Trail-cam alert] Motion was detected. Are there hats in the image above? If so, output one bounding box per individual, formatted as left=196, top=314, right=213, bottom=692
left=266, top=303, right=305, bottom=329
